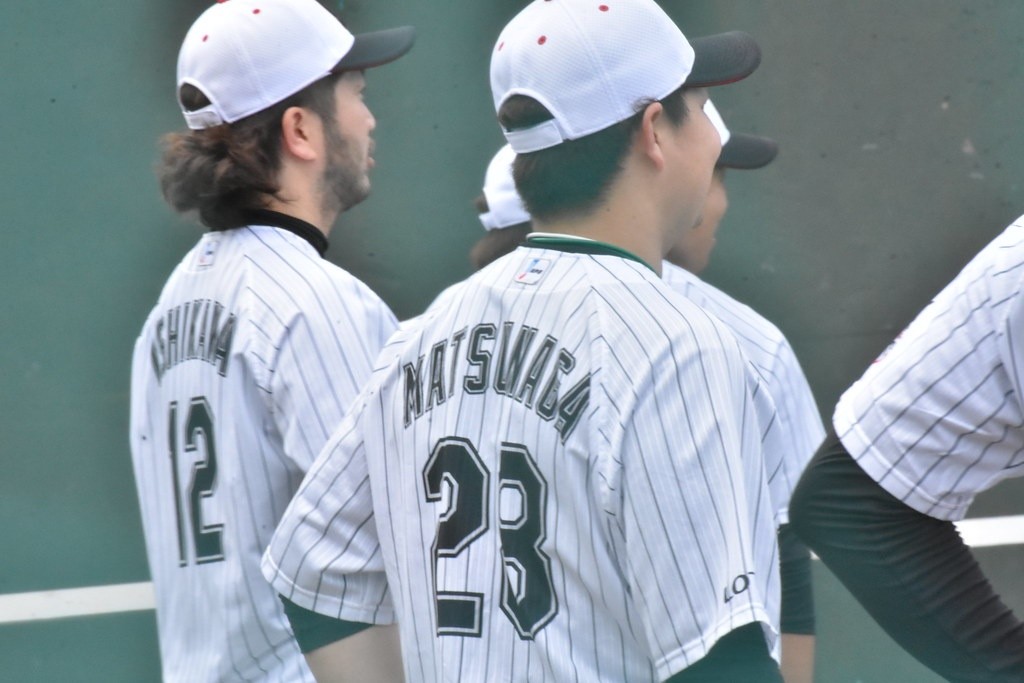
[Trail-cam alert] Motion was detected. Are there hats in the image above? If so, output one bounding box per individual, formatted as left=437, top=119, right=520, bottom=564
left=175, top=1, right=418, bottom=130
left=702, top=96, right=778, bottom=169
left=475, top=143, right=532, bottom=232
left=489, top=0, right=763, bottom=155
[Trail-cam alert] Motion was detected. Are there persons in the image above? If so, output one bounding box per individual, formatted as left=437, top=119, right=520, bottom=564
left=471, top=144, right=533, bottom=272
left=788, top=215, right=1024, bottom=683
left=661, top=98, right=828, bottom=683
left=256, top=0, right=785, bottom=683
left=128, top=0, right=400, bottom=683
